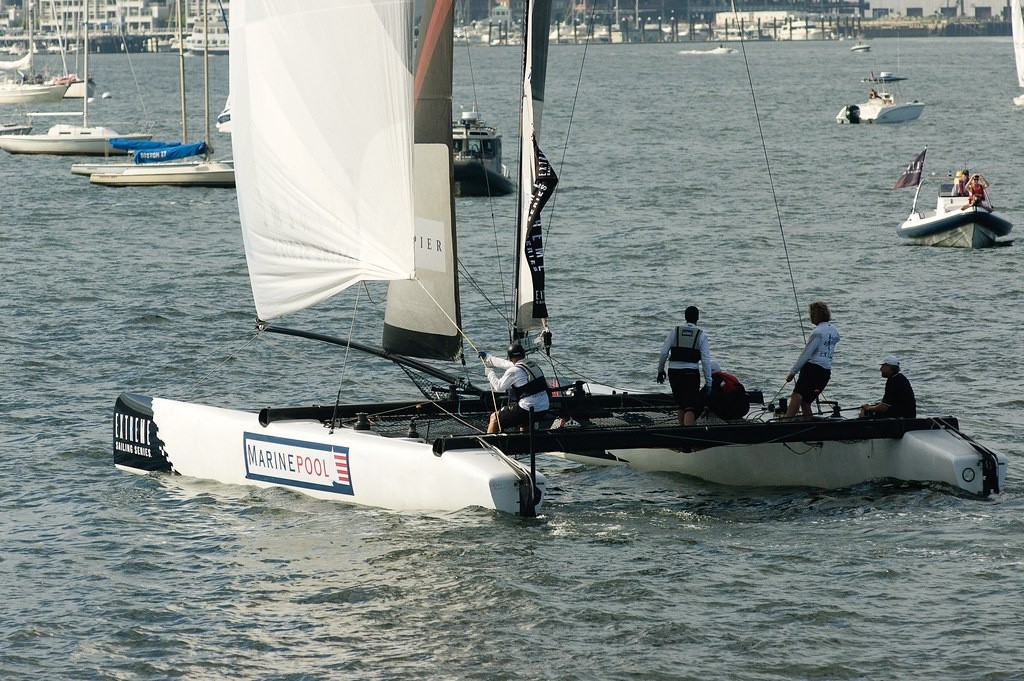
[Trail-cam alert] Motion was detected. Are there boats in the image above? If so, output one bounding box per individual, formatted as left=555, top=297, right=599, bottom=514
left=893, top=175, right=1015, bottom=250
left=861, top=32, right=909, bottom=82
left=450, top=101, right=521, bottom=201
left=835, top=78, right=925, bottom=124
left=850, top=45, right=871, bottom=53
left=712, top=46, right=734, bottom=53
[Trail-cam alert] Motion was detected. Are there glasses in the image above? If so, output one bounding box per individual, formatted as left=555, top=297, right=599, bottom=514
left=974, top=179, right=979, bottom=180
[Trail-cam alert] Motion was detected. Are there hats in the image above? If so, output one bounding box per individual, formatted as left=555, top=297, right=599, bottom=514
left=877, top=355, right=900, bottom=367
left=707, top=360, right=721, bottom=373
left=962, top=170, right=970, bottom=175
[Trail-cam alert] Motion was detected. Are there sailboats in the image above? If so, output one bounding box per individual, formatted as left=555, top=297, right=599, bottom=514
left=0, top=0, right=239, bottom=188
left=1009, top=0, right=1024, bottom=107
left=109, top=0, right=1008, bottom=524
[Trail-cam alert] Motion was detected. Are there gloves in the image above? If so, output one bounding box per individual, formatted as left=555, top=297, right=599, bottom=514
left=485, top=367, right=494, bottom=377
left=476, top=351, right=487, bottom=359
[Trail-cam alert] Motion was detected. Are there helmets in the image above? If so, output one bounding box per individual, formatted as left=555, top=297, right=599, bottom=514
left=506, top=343, right=526, bottom=361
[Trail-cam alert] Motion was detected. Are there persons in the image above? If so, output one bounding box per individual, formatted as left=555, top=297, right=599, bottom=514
left=869, top=72, right=876, bottom=80
left=477, top=343, right=550, bottom=434
left=951, top=169, right=970, bottom=196
left=869, top=88, right=877, bottom=98
left=859, top=356, right=918, bottom=419
left=783, top=302, right=841, bottom=422
left=699, top=360, right=750, bottom=421
left=656, top=306, right=714, bottom=426
left=965, top=174, right=989, bottom=204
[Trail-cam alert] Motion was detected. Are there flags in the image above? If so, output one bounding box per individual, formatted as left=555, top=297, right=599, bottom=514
left=892, top=149, right=926, bottom=190
